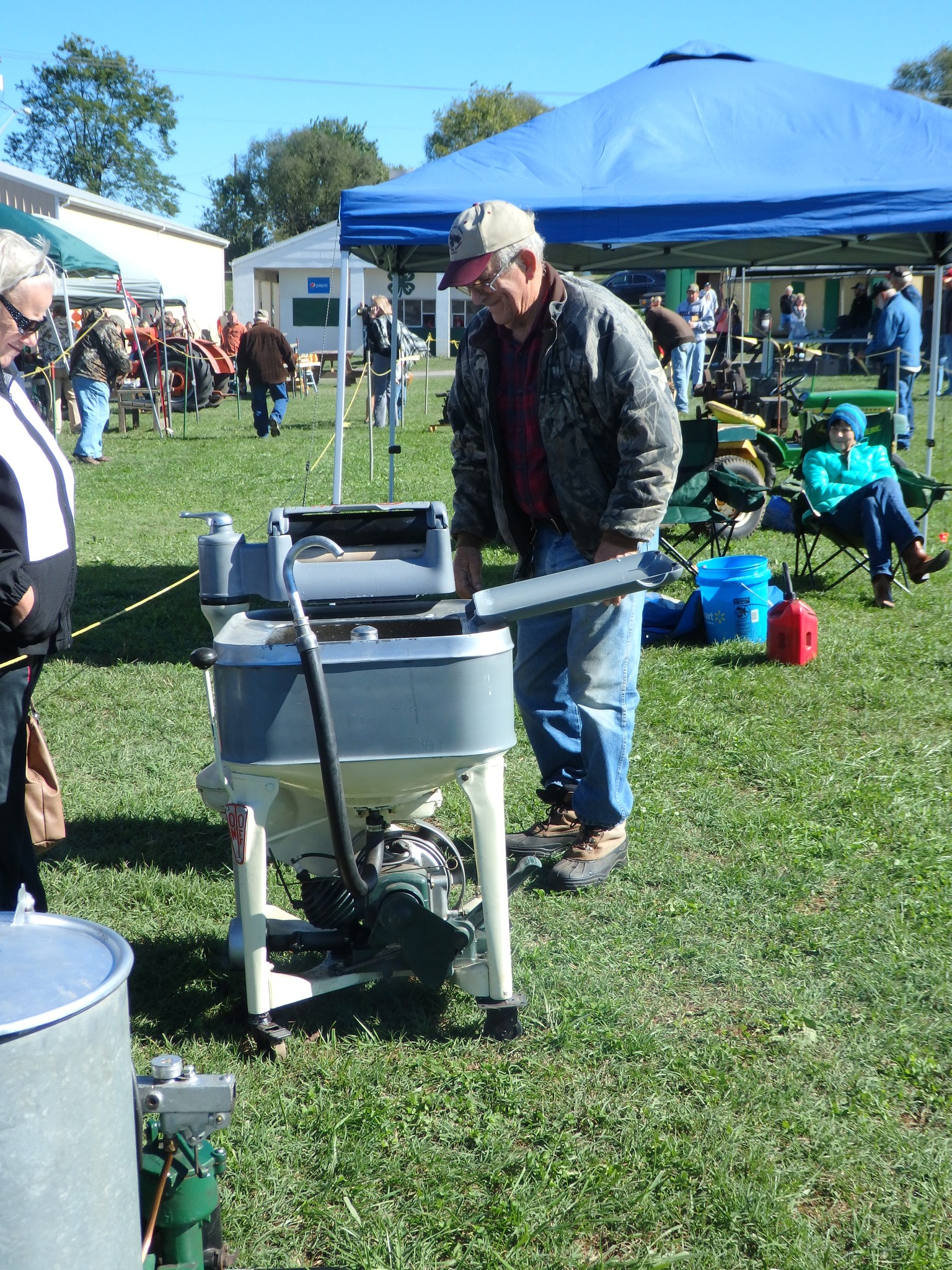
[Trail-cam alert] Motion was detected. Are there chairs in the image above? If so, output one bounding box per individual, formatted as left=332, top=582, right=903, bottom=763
left=777, top=407, right=952, bottom=595
left=660, top=405, right=771, bottom=575
left=116, top=370, right=174, bottom=433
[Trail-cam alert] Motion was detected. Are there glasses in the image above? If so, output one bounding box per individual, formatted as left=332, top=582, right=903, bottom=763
left=455, top=257, right=514, bottom=297
left=0, top=294, right=48, bottom=336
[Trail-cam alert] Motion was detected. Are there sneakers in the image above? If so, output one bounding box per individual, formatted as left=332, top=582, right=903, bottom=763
left=550, top=816, right=629, bottom=891
left=505, top=784, right=584, bottom=855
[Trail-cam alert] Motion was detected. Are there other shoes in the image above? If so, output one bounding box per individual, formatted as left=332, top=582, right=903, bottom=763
left=96, top=455, right=110, bottom=462
left=269, top=417, right=281, bottom=438
left=72, top=452, right=101, bottom=466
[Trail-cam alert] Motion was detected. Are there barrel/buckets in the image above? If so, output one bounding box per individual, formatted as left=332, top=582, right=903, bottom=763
left=752, top=308, right=772, bottom=337
left=0, top=882, right=141, bottom=1270
left=696, top=555, right=774, bottom=645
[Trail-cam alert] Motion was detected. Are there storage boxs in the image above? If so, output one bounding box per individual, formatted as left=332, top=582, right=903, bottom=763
left=817, top=360, right=839, bottom=376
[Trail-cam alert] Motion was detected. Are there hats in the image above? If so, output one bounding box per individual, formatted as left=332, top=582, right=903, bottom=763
left=255, top=309, right=269, bottom=319
left=686, top=283, right=699, bottom=292
left=108, top=315, right=128, bottom=339
left=867, top=281, right=893, bottom=300
left=827, top=403, right=868, bottom=440
left=705, top=282, right=711, bottom=286
left=884, top=265, right=911, bottom=277
left=438, top=199, right=536, bottom=291
left=852, top=282, right=865, bottom=290
left=164, top=311, right=172, bottom=318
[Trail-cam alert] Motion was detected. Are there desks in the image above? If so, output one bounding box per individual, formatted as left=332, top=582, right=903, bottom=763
left=283, top=362, right=320, bottom=398
left=776, top=338, right=873, bottom=374
left=307, top=351, right=357, bottom=387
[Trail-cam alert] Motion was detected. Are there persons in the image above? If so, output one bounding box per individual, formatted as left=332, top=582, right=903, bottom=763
left=802, top=403, right=950, bottom=608
left=0, top=230, right=78, bottom=915
left=14, top=294, right=408, bottom=464
left=644, top=265, right=952, bottom=450
left=436, top=200, right=684, bottom=890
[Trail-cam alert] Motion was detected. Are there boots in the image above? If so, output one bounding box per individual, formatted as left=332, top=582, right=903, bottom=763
left=902, top=538, right=950, bottom=585
left=872, top=573, right=895, bottom=608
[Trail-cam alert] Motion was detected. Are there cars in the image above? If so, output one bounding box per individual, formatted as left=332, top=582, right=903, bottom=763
left=599, top=269, right=667, bottom=304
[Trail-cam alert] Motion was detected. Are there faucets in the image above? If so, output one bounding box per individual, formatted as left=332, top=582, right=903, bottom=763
left=278, top=531, right=345, bottom=635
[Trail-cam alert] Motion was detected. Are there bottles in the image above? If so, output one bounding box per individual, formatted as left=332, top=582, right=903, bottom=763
left=767, top=563, right=817, bottom=664
left=300, top=353, right=318, bottom=363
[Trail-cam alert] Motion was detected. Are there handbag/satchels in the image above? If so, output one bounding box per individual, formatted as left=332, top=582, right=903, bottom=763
left=23, top=698, right=66, bottom=854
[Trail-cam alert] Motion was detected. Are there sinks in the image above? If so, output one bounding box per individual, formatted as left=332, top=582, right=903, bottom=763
left=259, top=617, right=461, bottom=639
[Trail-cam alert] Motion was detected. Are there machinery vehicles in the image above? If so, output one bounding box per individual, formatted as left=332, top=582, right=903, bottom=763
left=122, top=326, right=236, bottom=414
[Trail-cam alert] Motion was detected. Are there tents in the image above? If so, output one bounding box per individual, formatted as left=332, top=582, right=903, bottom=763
left=334, top=39, right=952, bottom=551
left=0, top=202, right=199, bottom=439
